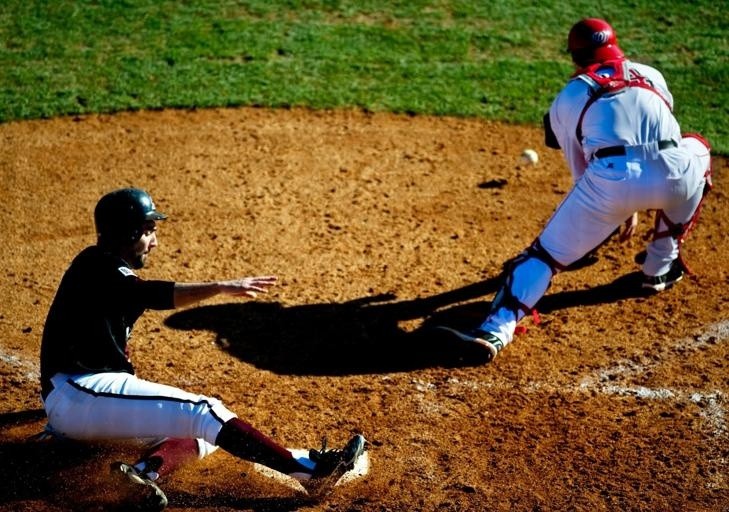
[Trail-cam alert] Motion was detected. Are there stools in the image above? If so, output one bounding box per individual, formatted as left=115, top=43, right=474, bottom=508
left=40, top=381, right=55, bottom=403
left=596, top=140, right=676, bottom=157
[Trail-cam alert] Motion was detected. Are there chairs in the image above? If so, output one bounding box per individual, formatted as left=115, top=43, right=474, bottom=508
left=641, top=263, right=684, bottom=293
left=307, top=434, right=365, bottom=504
left=426, top=323, right=504, bottom=364
left=108, top=462, right=168, bottom=510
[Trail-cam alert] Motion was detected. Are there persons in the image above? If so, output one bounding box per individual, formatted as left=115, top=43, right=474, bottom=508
left=436, top=18, right=711, bottom=361
left=39, top=188, right=365, bottom=507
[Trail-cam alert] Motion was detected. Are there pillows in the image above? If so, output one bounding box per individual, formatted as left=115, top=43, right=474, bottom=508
left=94, top=188, right=169, bottom=243
left=566, top=19, right=624, bottom=64
